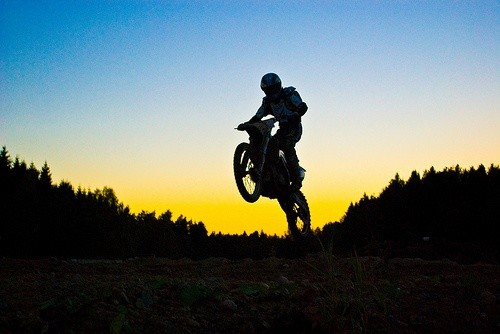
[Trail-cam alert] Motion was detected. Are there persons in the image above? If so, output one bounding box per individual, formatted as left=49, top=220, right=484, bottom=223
left=238, top=72, right=308, bottom=192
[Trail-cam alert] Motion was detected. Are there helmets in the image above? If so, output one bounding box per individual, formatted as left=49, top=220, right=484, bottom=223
left=260, top=73, right=281, bottom=93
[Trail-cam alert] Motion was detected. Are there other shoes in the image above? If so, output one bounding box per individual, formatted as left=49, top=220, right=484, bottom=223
left=289, top=183, right=302, bottom=194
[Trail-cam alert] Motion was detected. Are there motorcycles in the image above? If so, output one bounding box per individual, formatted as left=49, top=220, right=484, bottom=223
left=233, top=113, right=311, bottom=238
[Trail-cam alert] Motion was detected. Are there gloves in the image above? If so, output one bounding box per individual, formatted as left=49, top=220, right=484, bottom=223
left=237, top=124, right=246, bottom=131
left=284, top=113, right=296, bottom=122
